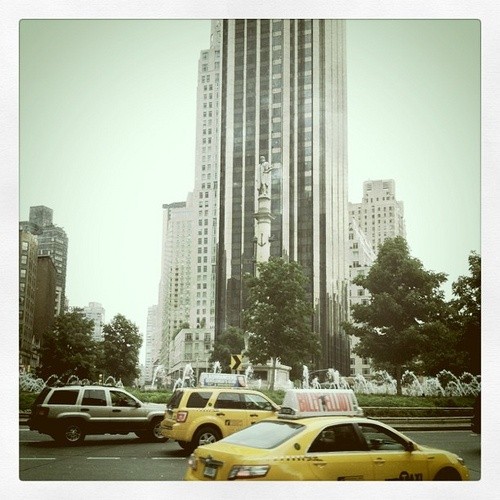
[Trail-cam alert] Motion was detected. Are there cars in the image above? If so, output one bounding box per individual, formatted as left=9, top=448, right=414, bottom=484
left=186, top=387, right=470, bottom=480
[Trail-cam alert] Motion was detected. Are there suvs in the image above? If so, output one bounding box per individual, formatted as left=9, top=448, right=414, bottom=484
left=158, top=387, right=287, bottom=452
left=27, top=384, right=173, bottom=442
